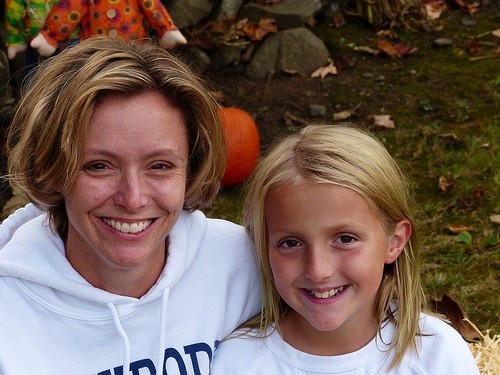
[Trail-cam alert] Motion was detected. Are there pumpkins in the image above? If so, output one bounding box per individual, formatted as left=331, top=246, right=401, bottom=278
left=216, top=106, right=260, bottom=186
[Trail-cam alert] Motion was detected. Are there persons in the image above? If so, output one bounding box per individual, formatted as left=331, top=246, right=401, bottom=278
left=211, top=124, right=479, bottom=375
left=0, top=35, right=280, bottom=375
